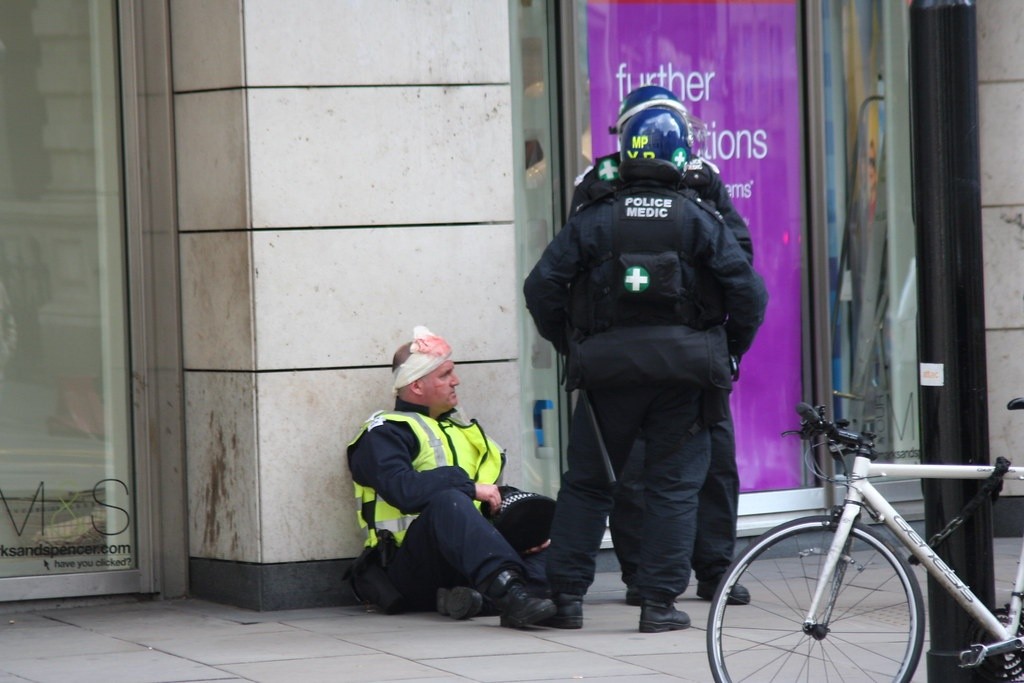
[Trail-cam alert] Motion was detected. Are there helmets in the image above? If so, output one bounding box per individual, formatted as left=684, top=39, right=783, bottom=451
left=616, top=85, right=690, bottom=133
left=620, top=106, right=691, bottom=182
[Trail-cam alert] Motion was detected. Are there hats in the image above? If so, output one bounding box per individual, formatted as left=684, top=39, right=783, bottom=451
left=481, top=483, right=555, bottom=548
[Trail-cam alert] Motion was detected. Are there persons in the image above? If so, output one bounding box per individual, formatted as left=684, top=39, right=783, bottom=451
left=347, top=325, right=558, bottom=629
left=523, top=86, right=769, bottom=632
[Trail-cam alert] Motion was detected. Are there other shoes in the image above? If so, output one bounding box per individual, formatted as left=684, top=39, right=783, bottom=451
left=696, top=577, right=750, bottom=606
left=535, top=590, right=584, bottom=630
left=433, top=581, right=492, bottom=619
left=638, top=602, right=691, bottom=632
left=626, top=587, right=644, bottom=605
left=484, top=569, right=559, bottom=629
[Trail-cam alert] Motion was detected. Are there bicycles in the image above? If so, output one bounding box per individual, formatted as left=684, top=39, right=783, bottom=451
left=707, top=401, right=1024, bottom=681
